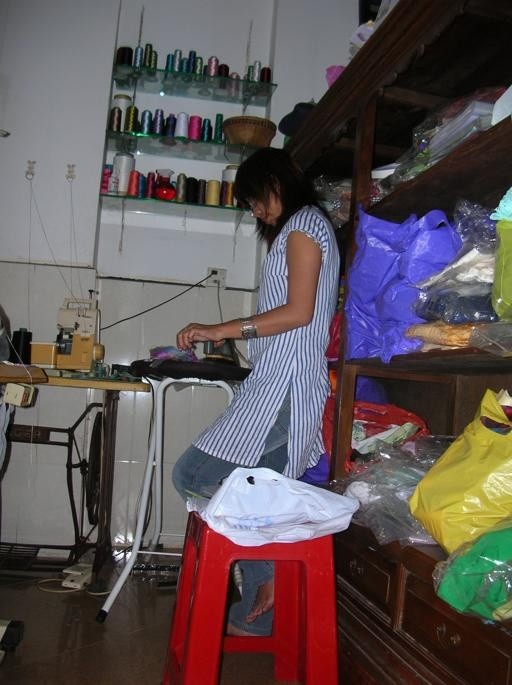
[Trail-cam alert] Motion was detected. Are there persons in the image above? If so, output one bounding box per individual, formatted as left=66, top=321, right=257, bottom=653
left=175, top=147, right=340, bottom=637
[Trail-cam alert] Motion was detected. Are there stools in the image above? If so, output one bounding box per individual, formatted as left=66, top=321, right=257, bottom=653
left=162, top=512, right=338, bottom=685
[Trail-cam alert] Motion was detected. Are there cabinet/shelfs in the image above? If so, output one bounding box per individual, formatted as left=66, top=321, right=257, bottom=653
left=276, top=0, right=512, bottom=679
left=98, top=63, right=279, bottom=224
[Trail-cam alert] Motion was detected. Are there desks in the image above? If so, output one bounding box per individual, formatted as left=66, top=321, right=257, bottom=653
left=95, top=365, right=252, bottom=625
left=8, top=373, right=151, bottom=596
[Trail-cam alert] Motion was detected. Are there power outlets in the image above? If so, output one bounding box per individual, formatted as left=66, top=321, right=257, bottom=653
left=206, top=267, right=228, bottom=288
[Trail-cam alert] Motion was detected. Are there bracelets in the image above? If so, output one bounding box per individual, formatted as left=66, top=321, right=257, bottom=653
left=238, top=314, right=259, bottom=342
